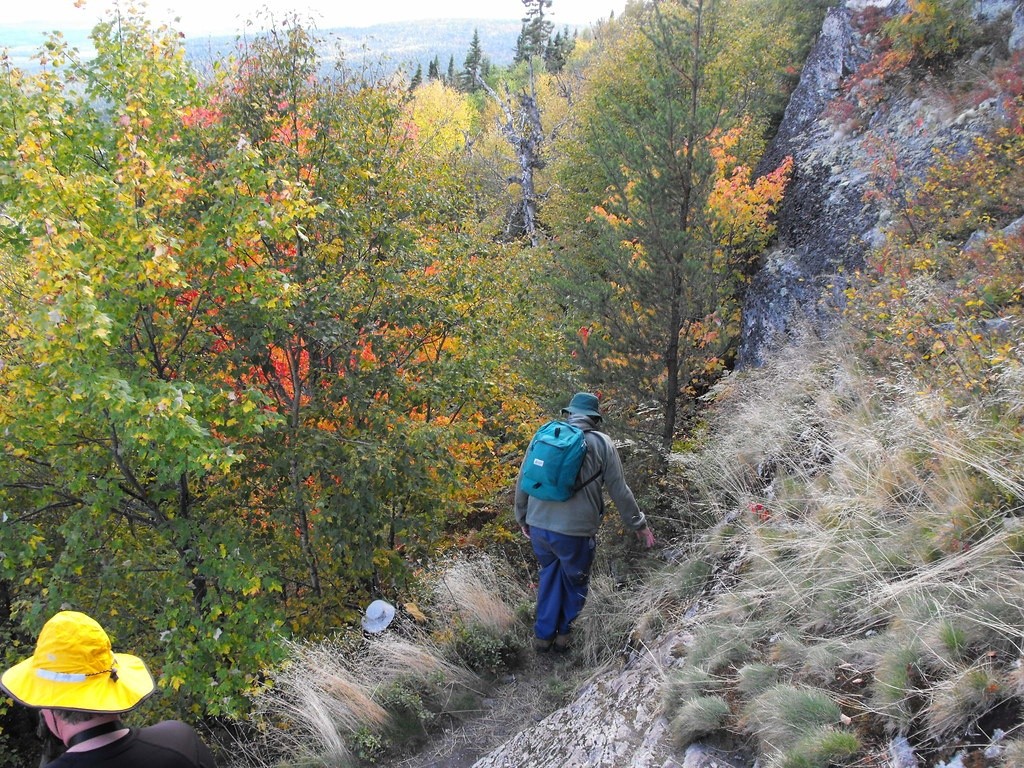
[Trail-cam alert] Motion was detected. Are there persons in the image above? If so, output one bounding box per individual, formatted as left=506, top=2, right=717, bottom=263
left=0, top=609, right=221, bottom=768
left=36, top=709, right=69, bottom=767
left=356, top=597, right=426, bottom=663
left=511, top=391, right=659, bottom=657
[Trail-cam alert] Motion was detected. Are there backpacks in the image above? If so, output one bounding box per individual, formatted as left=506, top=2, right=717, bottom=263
left=520, top=420, right=603, bottom=502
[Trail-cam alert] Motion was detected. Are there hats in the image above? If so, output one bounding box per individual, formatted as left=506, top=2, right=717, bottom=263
left=360, top=600, right=396, bottom=634
left=0, top=610, right=157, bottom=714
left=561, top=392, right=603, bottom=423
